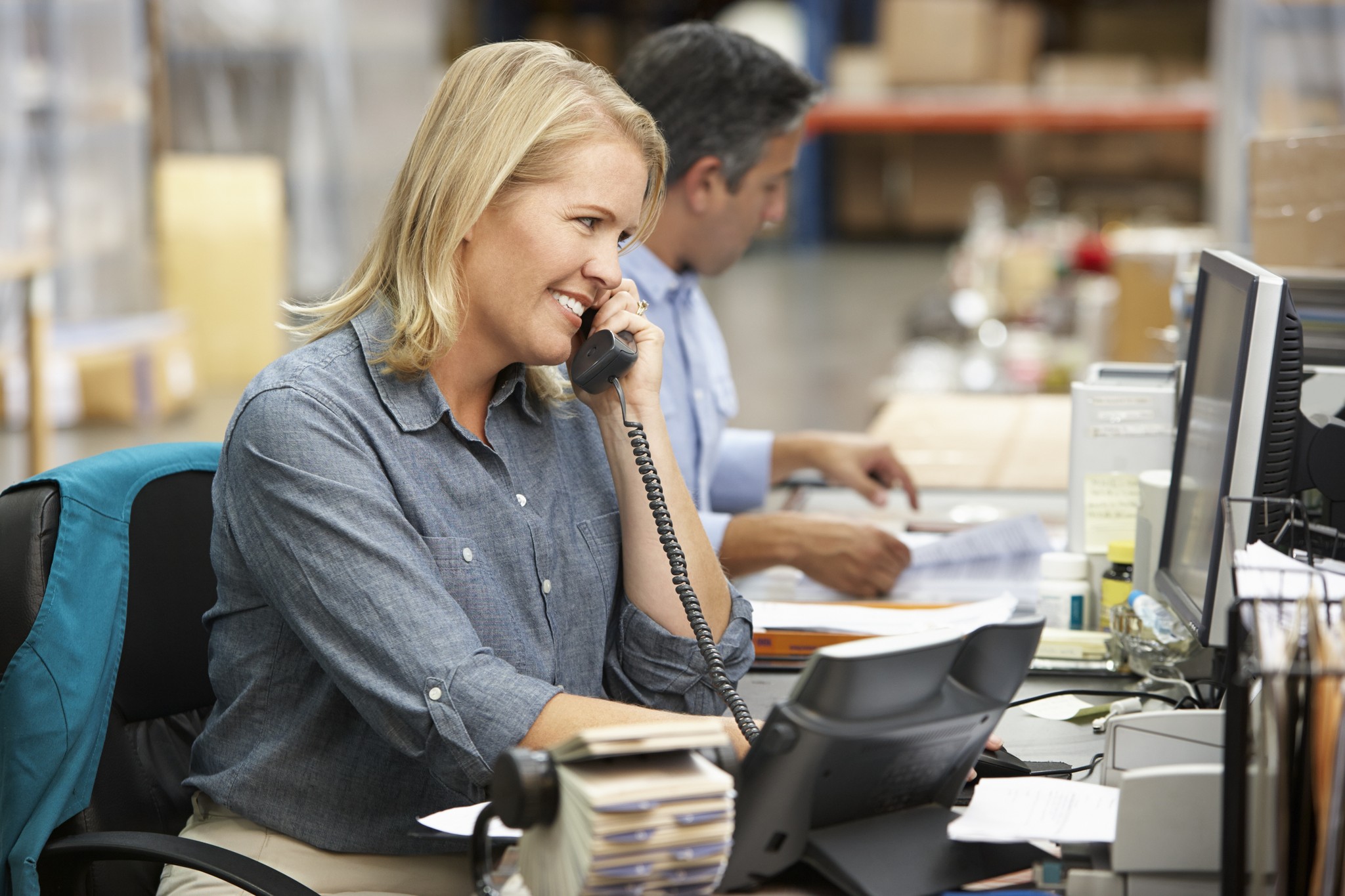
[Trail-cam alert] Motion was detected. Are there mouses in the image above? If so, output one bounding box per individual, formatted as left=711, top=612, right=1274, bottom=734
left=964, top=743, right=1031, bottom=786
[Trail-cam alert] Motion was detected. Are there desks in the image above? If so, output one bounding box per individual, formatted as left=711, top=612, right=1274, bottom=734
left=720, top=381, right=1123, bottom=896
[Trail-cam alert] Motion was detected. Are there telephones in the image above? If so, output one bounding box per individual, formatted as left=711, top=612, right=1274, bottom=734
left=565, top=305, right=636, bottom=398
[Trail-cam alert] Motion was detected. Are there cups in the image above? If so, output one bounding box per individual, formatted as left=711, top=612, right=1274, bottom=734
left=1132, top=469, right=1199, bottom=601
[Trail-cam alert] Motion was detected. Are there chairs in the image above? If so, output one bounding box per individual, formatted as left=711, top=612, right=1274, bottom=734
left=0, top=445, right=318, bottom=896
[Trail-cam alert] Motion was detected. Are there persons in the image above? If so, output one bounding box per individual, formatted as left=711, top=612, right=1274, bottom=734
left=554, top=21, right=922, bottom=599
left=155, top=36, right=1005, bottom=896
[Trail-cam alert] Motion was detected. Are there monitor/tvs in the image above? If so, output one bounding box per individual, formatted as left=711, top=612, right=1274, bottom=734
left=1147, top=248, right=1344, bottom=648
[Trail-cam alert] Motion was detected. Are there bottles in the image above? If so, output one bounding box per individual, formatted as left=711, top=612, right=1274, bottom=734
left=1099, top=538, right=1136, bottom=632
left=1037, top=552, right=1091, bottom=630
